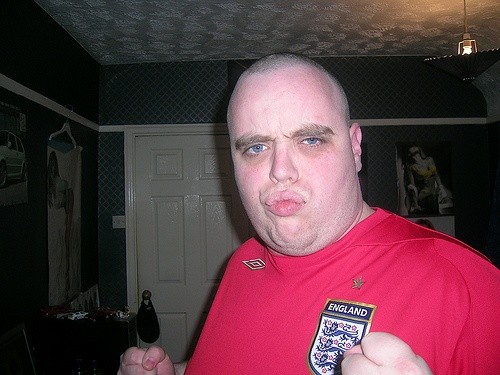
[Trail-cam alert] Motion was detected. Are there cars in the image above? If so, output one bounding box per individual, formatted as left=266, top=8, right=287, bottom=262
left=0, top=129, right=27, bottom=188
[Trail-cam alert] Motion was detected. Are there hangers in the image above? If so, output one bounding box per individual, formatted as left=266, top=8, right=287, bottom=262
left=47, top=103, right=78, bottom=152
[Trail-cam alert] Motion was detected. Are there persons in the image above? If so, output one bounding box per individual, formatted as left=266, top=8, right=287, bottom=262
left=48, top=151, right=74, bottom=279
left=405, top=146, right=453, bottom=217
left=118, top=54, right=500, bottom=374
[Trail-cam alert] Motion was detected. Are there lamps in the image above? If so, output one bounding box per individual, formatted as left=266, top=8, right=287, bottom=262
left=458, top=0, right=478, bottom=55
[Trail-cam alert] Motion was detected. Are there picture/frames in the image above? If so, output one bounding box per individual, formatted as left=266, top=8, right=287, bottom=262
left=0, top=323, right=42, bottom=375
